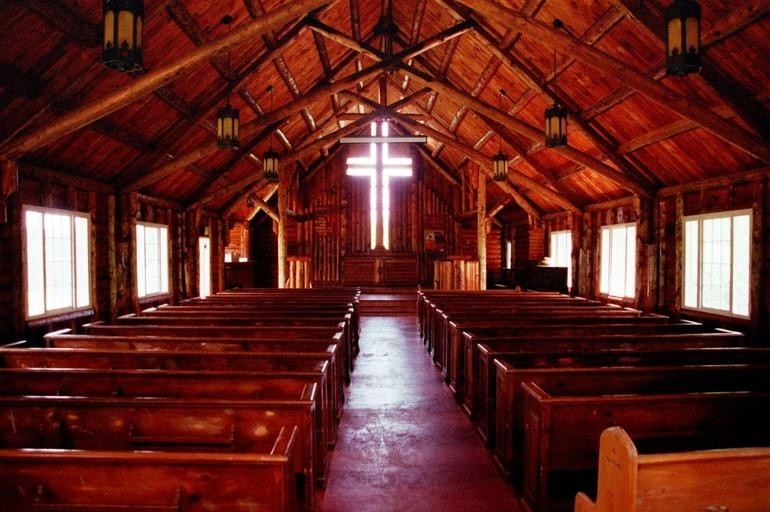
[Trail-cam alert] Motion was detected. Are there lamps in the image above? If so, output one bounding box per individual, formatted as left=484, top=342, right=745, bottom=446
left=661, top=1, right=704, bottom=77
left=215, top=14, right=280, bottom=180
left=490, top=21, right=569, bottom=180
left=99, top=0, right=145, bottom=75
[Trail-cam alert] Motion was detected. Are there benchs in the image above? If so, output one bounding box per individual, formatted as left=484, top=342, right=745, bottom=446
left=417, top=288, right=770, bottom=512
left=1, top=284, right=362, bottom=510
